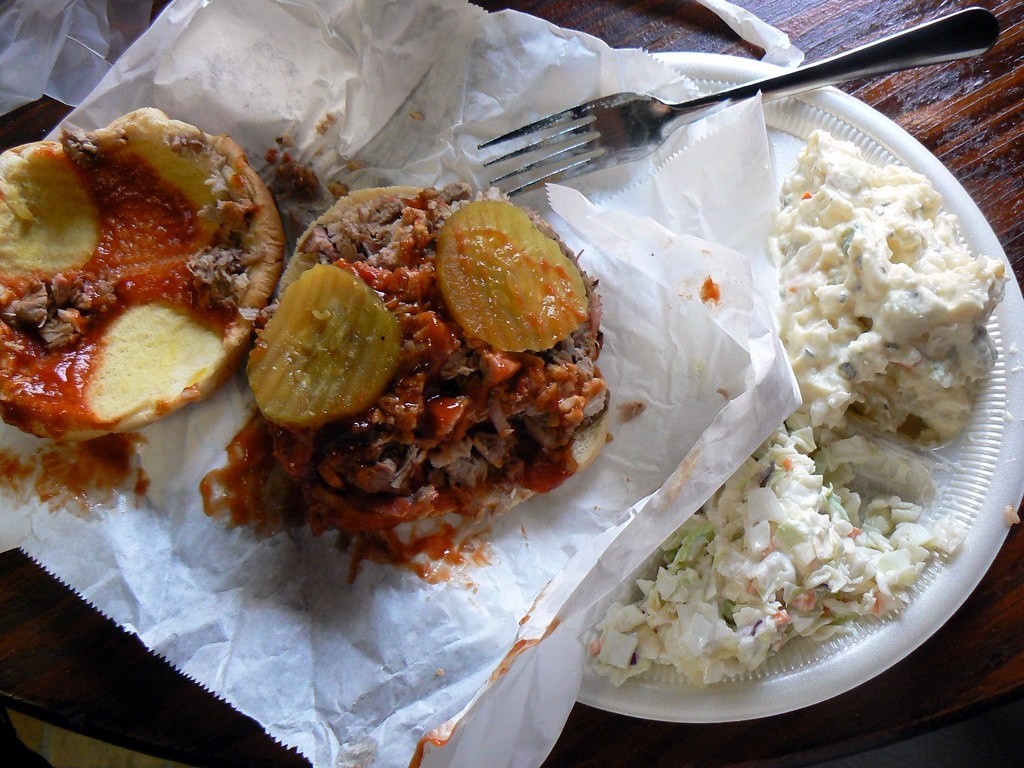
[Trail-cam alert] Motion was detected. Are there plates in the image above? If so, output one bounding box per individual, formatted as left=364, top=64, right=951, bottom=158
left=576, top=51, right=1024, bottom=722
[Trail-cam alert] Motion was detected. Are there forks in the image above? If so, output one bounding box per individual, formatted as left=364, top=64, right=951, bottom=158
left=477, top=5, right=1003, bottom=202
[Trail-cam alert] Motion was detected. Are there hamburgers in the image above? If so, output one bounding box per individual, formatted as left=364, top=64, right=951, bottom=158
left=0, top=105, right=613, bottom=535
